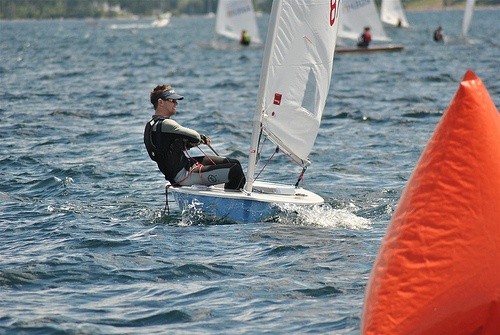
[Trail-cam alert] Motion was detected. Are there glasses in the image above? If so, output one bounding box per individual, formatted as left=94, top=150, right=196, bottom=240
left=157, top=97, right=176, bottom=103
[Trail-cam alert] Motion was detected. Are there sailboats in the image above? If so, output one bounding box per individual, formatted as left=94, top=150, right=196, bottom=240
left=334, top=0, right=404, bottom=53
left=380, top=0, right=411, bottom=29
left=212, top=0, right=263, bottom=52
left=165, top=0, right=341, bottom=226
left=454, top=0, right=480, bottom=46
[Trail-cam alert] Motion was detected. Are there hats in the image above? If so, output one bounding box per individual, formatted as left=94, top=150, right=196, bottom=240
left=153, top=90, right=184, bottom=105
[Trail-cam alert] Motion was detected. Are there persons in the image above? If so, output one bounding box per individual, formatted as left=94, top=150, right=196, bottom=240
left=144, top=84, right=247, bottom=192
left=356, top=27, right=372, bottom=48
left=434, top=27, right=444, bottom=43
left=240, top=30, right=250, bottom=46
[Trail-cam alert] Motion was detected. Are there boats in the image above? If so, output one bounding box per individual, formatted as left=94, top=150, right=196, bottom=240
left=150, top=12, right=172, bottom=29
